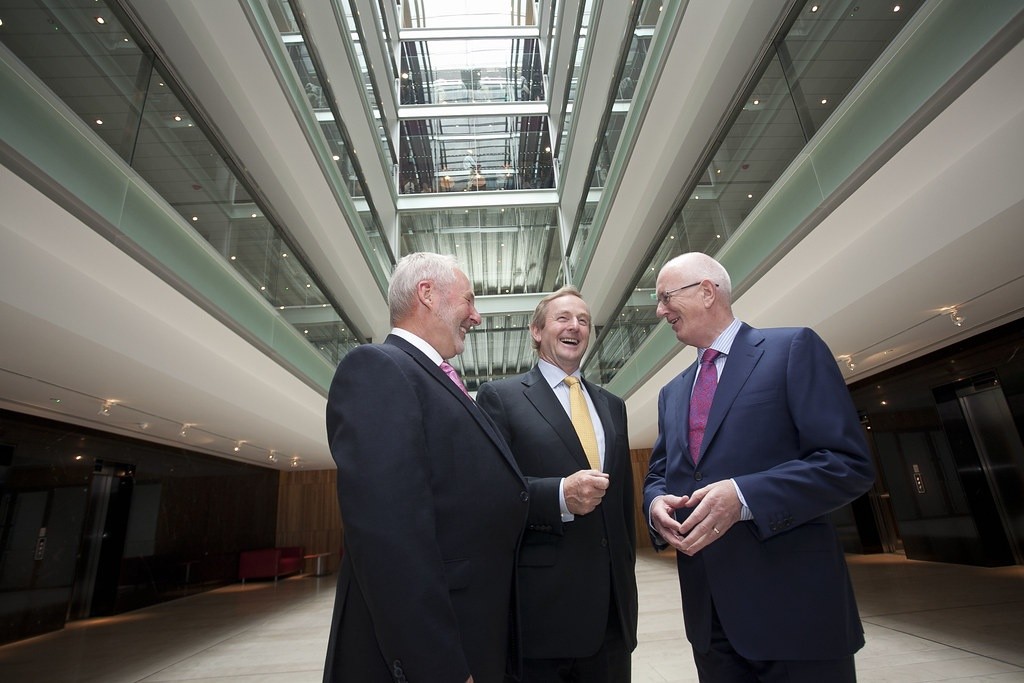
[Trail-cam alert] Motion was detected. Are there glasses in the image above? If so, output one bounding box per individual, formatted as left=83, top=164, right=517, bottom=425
left=656, top=280, right=721, bottom=306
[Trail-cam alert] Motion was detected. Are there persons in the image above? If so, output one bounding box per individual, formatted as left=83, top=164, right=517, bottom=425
left=641, top=252, right=877, bottom=683
left=322, top=252, right=531, bottom=683
left=516, top=71, right=528, bottom=101
left=462, top=150, right=476, bottom=169
left=476, top=286, right=638, bottom=683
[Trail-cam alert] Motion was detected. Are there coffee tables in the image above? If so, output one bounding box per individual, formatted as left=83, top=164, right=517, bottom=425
left=304, top=552, right=333, bottom=576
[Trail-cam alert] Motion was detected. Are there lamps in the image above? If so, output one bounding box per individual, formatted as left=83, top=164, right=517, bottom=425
left=97, top=399, right=301, bottom=469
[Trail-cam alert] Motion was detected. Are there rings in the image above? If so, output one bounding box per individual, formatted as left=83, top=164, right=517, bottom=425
left=712, top=527, right=720, bottom=534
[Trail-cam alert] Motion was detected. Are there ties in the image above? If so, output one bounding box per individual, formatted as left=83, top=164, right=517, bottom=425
left=561, top=375, right=602, bottom=474
left=440, top=358, right=475, bottom=406
left=686, top=349, right=720, bottom=468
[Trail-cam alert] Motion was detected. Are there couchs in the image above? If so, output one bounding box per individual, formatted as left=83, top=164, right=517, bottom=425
left=239, top=546, right=306, bottom=585
